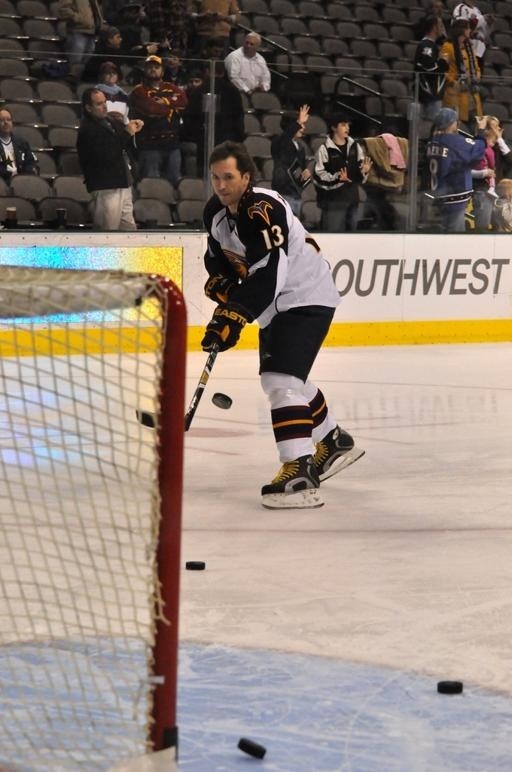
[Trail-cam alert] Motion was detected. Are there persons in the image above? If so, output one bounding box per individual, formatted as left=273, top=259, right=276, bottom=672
left=200, top=141, right=354, bottom=497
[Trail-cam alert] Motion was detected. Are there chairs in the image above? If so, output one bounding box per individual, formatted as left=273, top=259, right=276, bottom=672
left=0, top=0, right=512, bottom=232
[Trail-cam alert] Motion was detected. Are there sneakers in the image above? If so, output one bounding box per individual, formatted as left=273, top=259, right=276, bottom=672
left=260, top=454, right=320, bottom=494
left=312, top=425, right=354, bottom=475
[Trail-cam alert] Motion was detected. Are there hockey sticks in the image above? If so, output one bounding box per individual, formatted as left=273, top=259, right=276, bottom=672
left=136, top=343, right=220, bottom=434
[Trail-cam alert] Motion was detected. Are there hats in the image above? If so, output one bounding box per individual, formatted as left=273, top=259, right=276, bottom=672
left=433, top=107, right=458, bottom=131
left=145, top=54, right=162, bottom=66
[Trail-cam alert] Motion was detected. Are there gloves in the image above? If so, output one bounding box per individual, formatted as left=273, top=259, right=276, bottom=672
left=201, top=306, right=247, bottom=355
left=206, top=274, right=240, bottom=306
